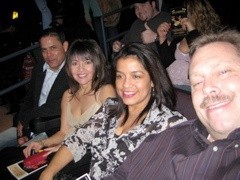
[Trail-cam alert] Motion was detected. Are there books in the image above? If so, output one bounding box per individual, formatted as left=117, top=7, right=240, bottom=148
left=6, top=158, right=48, bottom=180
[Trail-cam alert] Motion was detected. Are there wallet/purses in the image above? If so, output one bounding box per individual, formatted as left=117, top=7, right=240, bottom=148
left=23, top=149, right=51, bottom=169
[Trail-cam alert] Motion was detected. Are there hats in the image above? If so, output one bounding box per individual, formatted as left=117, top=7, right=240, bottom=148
left=130, top=0, right=149, bottom=9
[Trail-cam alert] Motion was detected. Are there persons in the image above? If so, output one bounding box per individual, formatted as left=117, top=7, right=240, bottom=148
left=0, top=28, right=240, bottom=180
left=112, top=0, right=221, bottom=90
